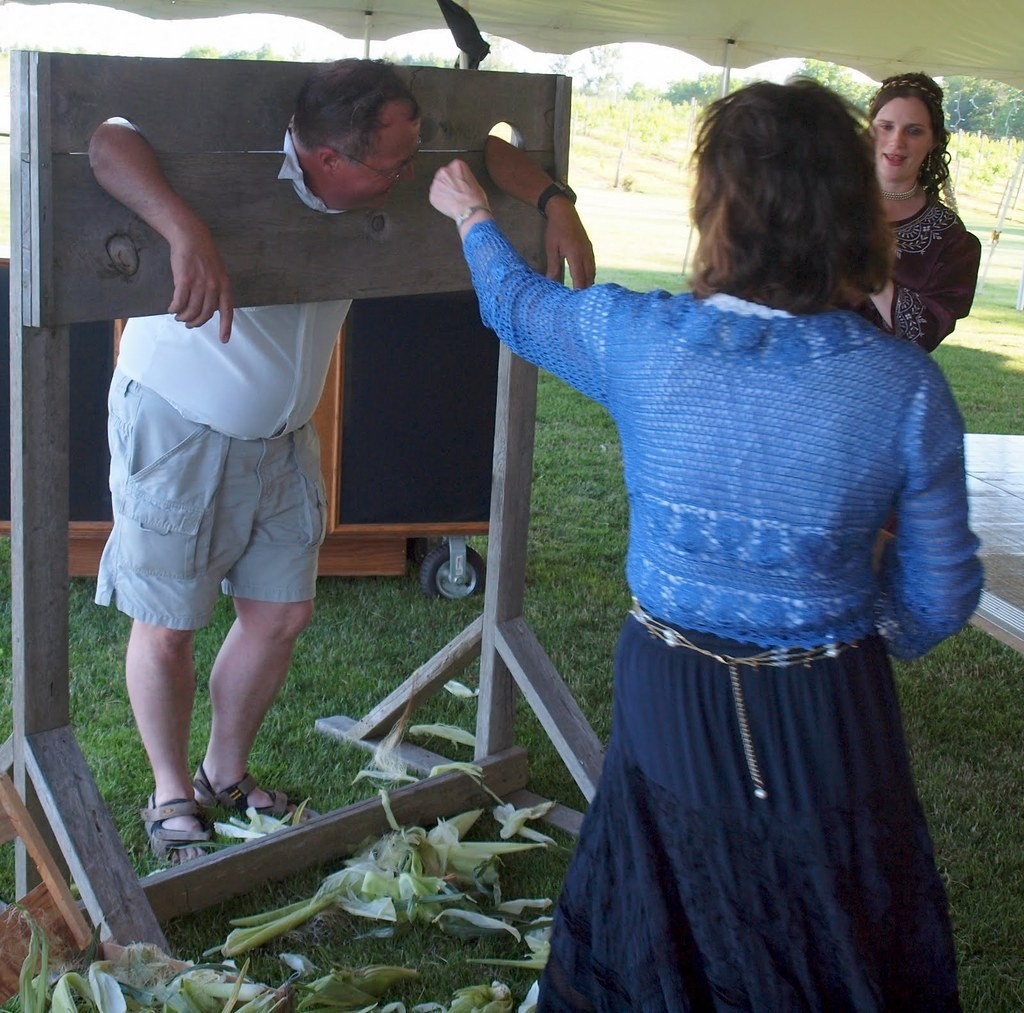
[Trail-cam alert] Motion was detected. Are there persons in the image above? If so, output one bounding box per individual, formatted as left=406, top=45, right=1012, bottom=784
left=428, top=77, right=988, bottom=1013
left=865, top=73, right=981, bottom=352
left=93, top=58, right=595, bottom=874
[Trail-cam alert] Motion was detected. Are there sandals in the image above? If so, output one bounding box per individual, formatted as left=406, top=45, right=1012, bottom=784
left=140, top=788, right=217, bottom=872
left=190, top=761, right=321, bottom=840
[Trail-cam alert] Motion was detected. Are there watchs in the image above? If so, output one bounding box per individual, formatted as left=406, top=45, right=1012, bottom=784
left=538, top=180, right=577, bottom=219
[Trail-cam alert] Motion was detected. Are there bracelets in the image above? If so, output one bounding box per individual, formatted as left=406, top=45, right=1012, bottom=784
left=455, top=206, right=496, bottom=228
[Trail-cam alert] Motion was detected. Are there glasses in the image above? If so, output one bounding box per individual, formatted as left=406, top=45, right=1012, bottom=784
left=317, top=135, right=422, bottom=184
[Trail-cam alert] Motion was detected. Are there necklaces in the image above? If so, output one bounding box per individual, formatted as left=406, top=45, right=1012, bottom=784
left=881, top=182, right=920, bottom=200
